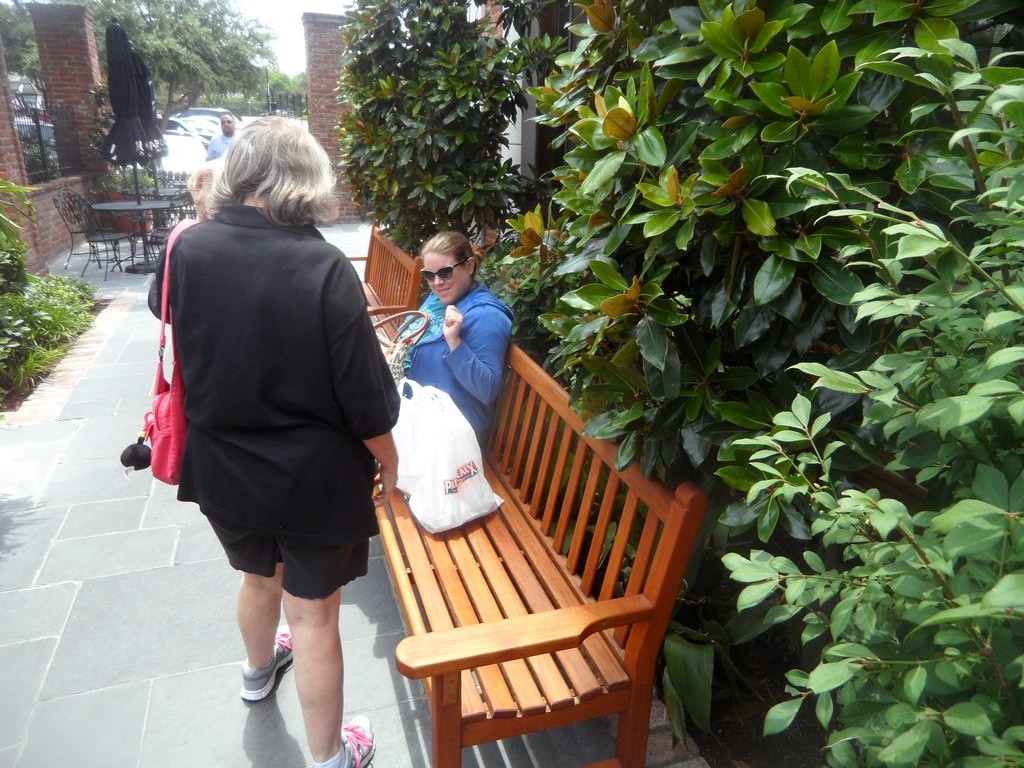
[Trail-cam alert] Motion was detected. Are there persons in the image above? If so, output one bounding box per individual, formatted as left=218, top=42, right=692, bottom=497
left=206, top=111, right=238, bottom=164
left=150, top=117, right=402, bottom=768
left=383, top=229, right=518, bottom=458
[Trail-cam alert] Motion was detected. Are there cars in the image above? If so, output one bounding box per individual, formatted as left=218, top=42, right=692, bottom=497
left=123, top=118, right=208, bottom=176
left=175, top=107, right=239, bottom=142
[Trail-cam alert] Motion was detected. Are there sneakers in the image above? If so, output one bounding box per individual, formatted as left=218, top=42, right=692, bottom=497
left=239, top=624, right=294, bottom=702
left=340, top=715, right=376, bottom=768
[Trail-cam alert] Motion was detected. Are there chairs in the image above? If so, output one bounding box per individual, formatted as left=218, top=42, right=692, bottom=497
left=53, top=194, right=102, bottom=270
left=151, top=191, right=198, bottom=248
left=60, top=187, right=136, bottom=281
left=100, top=181, right=147, bottom=251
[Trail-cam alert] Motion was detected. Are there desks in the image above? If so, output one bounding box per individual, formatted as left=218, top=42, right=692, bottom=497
left=116, top=186, right=189, bottom=245
left=90, top=199, right=190, bottom=273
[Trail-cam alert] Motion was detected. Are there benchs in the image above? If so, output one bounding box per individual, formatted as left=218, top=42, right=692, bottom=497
left=372, top=338, right=708, bottom=768
left=346, top=219, right=425, bottom=344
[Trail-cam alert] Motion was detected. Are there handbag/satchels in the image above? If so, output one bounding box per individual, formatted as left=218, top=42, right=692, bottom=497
left=142, top=216, right=198, bottom=485
left=386, top=378, right=506, bottom=534
left=372, top=311, right=431, bottom=389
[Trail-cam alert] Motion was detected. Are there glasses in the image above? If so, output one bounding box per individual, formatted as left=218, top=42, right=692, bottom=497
left=420, top=256, right=471, bottom=281
left=221, top=120, right=235, bottom=124
